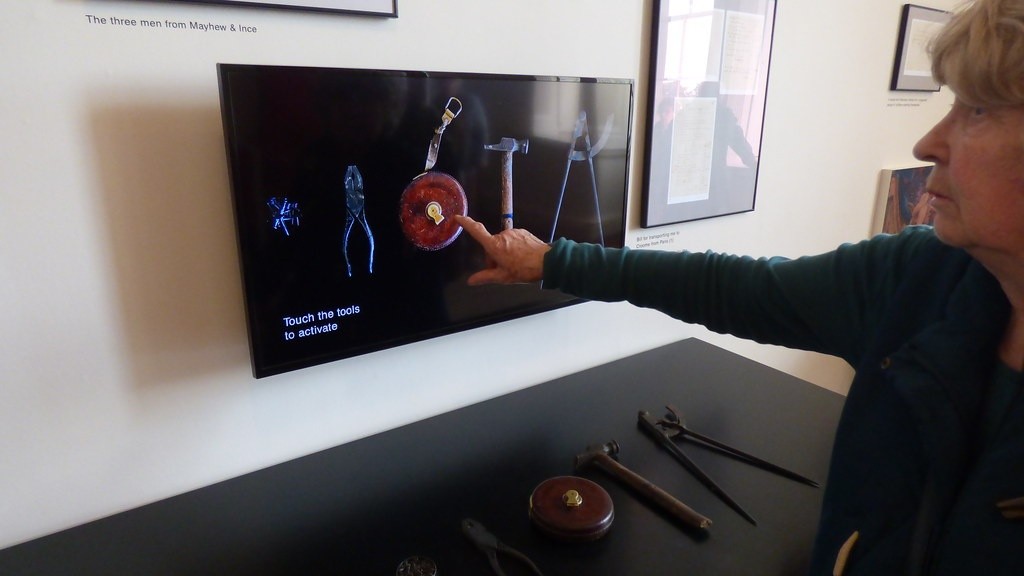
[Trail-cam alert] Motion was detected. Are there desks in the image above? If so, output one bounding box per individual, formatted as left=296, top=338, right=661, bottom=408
left=0, top=337, right=847, bottom=576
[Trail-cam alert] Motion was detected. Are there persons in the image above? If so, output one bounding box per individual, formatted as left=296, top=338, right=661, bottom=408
left=450, top=1, right=1024, bottom=575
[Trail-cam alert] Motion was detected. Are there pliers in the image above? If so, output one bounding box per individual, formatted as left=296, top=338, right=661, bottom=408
left=343, top=165, right=376, bottom=278
left=461, top=518, right=541, bottom=576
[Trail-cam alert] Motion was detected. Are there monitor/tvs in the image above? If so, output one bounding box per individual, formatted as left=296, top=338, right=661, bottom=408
left=216, top=64, right=630, bottom=379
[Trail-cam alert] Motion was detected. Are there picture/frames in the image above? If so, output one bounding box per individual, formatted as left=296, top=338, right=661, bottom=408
left=641, top=1, right=777, bottom=228
left=892, top=4, right=956, bottom=92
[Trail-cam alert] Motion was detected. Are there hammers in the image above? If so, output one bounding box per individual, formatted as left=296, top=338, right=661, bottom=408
left=484, top=137, right=529, bottom=231
left=573, top=439, right=713, bottom=531
